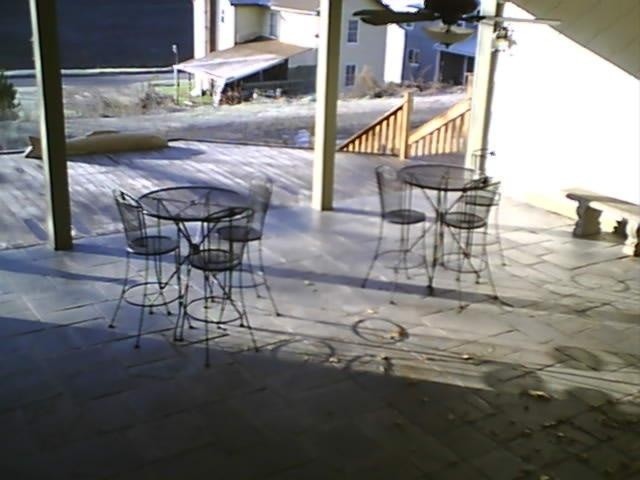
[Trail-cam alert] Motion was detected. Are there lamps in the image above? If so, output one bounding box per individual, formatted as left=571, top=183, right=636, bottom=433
left=423, top=18, right=476, bottom=49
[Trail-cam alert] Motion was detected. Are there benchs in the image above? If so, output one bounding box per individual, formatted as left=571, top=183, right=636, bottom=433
left=566, top=188, right=640, bottom=258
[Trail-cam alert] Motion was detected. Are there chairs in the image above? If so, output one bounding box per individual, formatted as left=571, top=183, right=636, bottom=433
left=109, top=174, right=280, bottom=367
left=362, top=160, right=501, bottom=295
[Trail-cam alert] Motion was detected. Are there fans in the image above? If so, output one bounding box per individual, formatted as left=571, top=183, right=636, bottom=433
left=351, top=0, right=565, bottom=31
